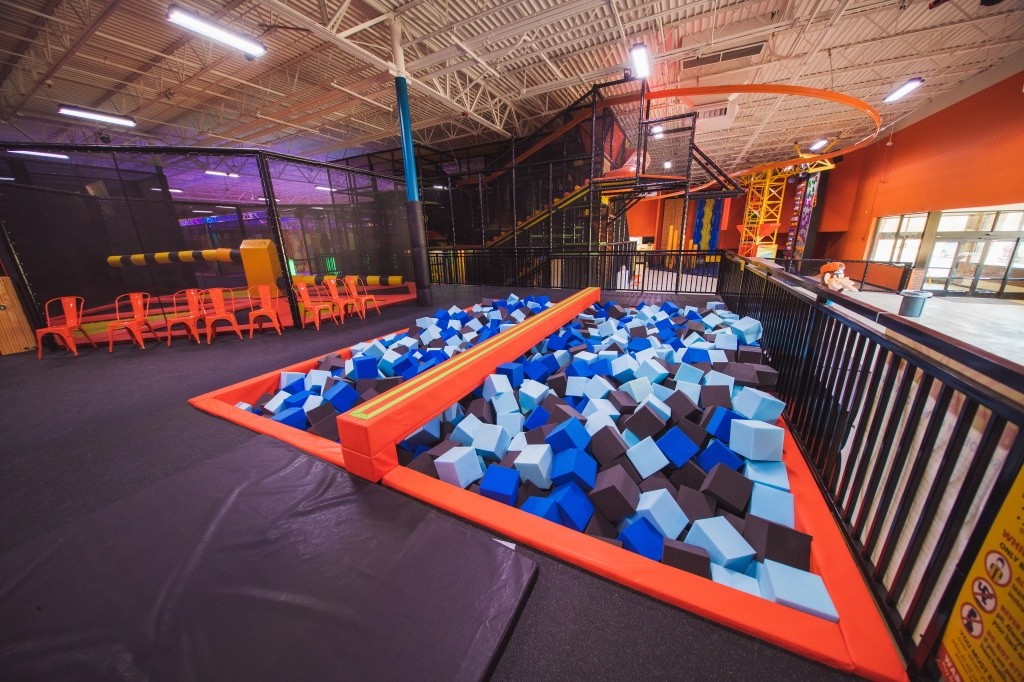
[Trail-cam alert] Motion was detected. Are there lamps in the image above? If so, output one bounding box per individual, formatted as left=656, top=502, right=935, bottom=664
left=629, top=41, right=650, bottom=79
left=166, top=2, right=268, bottom=61
left=57, top=103, right=136, bottom=128
left=882, top=76, right=925, bottom=103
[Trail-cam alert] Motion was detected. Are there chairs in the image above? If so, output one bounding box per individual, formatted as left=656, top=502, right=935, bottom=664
left=36, top=296, right=99, bottom=360
left=292, top=281, right=339, bottom=331
left=342, top=275, right=381, bottom=319
left=108, top=291, right=162, bottom=352
left=166, top=288, right=216, bottom=347
left=248, top=284, right=285, bottom=338
left=320, top=279, right=362, bottom=324
left=195, top=288, right=244, bottom=345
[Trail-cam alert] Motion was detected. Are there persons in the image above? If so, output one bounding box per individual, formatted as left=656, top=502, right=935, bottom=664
left=820, top=261, right=859, bottom=295
